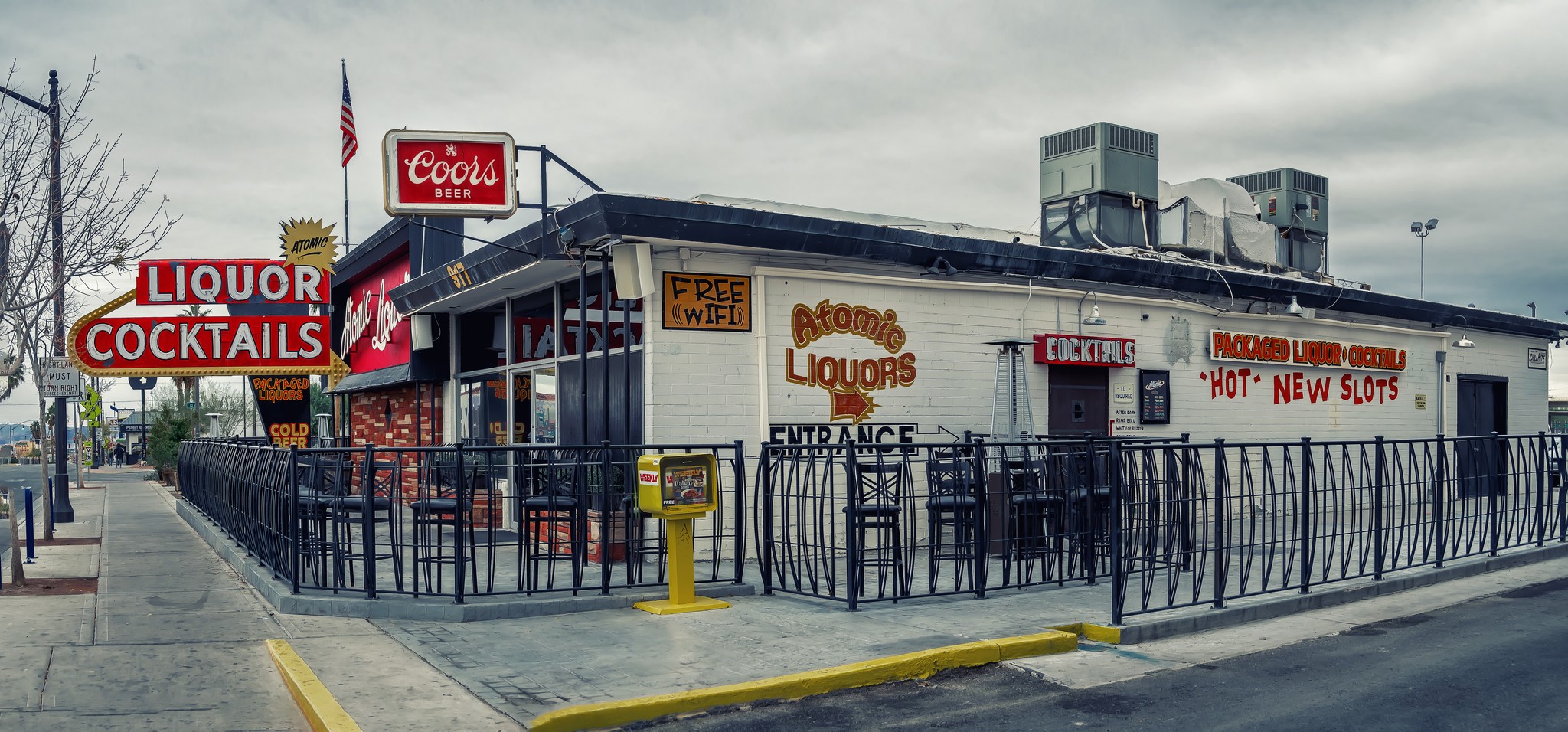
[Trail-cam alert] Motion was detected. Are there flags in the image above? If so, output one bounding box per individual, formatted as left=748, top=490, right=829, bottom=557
left=339, top=63, right=359, bottom=169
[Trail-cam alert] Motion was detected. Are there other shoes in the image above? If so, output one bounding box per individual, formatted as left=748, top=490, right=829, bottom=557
left=114, top=466, right=117, bottom=468
left=108, top=464, right=113, bottom=466
left=120, top=466, right=121, bottom=468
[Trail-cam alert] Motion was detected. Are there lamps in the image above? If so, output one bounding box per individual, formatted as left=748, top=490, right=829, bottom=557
left=1284, top=295, right=1303, bottom=314
left=1432, top=315, right=1476, bottom=349
left=927, top=256, right=957, bottom=277
left=1555, top=341, right=1560, bottom=348
left=1550, top=340, right=1554, bottom=344
left=1079, top=291, right=1108, bottom=336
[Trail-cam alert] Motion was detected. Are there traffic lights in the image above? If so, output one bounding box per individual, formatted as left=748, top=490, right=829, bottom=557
left=83, top=446, right=84, bottom=450
left=88, top=438, right=91, bottom=442
left=73, top=437, right=75, bottom=443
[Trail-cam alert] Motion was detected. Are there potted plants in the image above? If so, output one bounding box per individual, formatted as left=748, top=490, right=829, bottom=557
left=464, top=455, right=488, bottom=490
left=587, top=464, right=623, bottom=511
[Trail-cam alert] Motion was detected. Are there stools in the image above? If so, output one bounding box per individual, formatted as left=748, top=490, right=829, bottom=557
left=329, top=459, right=401, bottom=593
left=842, top=462, right=905, bottom=598
left=522, top=458, right=580, bottom=597
left=623, top=456, right=669, bottom=589
left=1063, top=454, right=1124, bottom=578
left=926, top=462, right=978, bottom=595
left=1002, top=458, right=1065, bottom=589
left=280, top=456, right=355, bottom=588
left=410, top=461, right=478, bottom=599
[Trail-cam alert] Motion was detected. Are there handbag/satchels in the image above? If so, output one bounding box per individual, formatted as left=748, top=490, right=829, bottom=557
left=109, top=452, right=113, bottom=457
left=123, top=455, right=126, bottom=463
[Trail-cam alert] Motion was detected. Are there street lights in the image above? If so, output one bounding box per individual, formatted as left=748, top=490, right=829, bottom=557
left=10, top=424, right=25, bottom=457
left=1411, top=218, right=1439, bottom=300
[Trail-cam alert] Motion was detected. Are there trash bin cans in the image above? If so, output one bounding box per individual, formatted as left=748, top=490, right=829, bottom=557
left=988, top=469, right=1045, bottom=562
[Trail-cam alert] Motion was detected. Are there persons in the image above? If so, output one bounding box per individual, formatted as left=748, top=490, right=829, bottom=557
left=108, top=443, right=124, bottom=468
